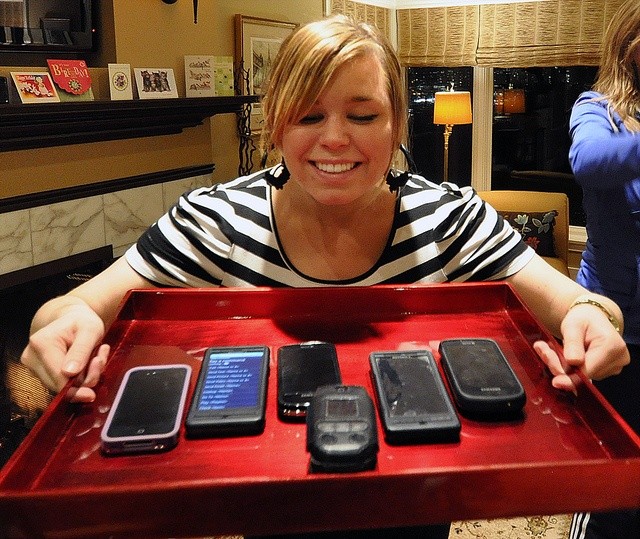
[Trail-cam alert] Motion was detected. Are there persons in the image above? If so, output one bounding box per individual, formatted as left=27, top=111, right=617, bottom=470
left=567, top=0, right=639, bottom=538
left=17, top=13, right=636, bottom=402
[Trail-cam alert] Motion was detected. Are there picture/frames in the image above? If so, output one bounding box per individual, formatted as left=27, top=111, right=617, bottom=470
left=234, top=13, right=302, bottom=142
left=134, top=68, right=178, bottom=99
left=10, top=70, right=60, bottom=104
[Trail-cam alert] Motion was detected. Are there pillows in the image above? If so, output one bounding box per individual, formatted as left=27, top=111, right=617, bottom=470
left=498, top=210, right=557, bottom=255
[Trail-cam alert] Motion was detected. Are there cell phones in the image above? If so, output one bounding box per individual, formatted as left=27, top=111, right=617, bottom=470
left=277, top=342, right=345, bottom=424
left=439, top=338, right=527, bottom=423
left=185, top=345, right=270, bottom=437
left=100, top=364, right=192, bottom=454
left=305, top=384, right=379, bottom=473
left=368, top=349, right=461, bottom=445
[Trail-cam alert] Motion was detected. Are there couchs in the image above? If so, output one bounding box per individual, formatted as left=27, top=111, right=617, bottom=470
left=476, top=190, right=570, bottom=278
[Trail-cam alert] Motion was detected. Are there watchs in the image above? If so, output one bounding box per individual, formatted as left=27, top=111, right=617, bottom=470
left=559, top=299, right=624, bottom=334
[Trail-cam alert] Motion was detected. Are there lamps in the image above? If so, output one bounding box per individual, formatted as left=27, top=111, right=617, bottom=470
left=494, top=86, right=526, bottom=114
left=434, top=87, right=471, bottom=182
